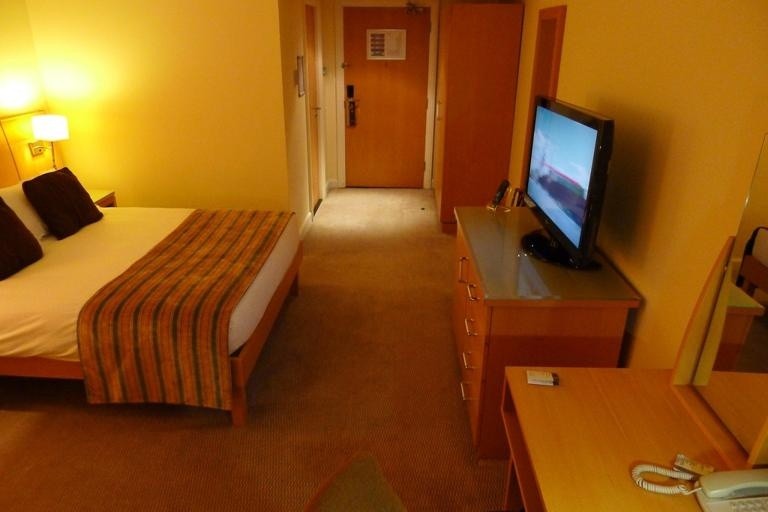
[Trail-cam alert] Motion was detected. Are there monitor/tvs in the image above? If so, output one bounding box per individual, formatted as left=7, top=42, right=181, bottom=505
left=521, top=95, right=614, bottom=270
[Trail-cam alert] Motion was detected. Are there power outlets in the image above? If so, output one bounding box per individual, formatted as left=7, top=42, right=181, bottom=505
left=30, top=141, right=46, bottom=154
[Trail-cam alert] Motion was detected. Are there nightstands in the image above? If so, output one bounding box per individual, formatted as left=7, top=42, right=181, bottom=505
left=87, top=190, right=119, bottom=207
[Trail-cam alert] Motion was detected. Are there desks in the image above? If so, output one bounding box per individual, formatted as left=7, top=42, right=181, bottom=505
left=500, top=366, right=728, bottom=512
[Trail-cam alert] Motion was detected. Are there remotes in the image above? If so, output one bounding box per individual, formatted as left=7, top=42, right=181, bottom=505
left=489, top=180, right=508, bottom=208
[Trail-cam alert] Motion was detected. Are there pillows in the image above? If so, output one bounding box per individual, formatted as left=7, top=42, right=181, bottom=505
left=1, top=195, right=43, bottom=281
left=22, top=167, right=103, bottom=240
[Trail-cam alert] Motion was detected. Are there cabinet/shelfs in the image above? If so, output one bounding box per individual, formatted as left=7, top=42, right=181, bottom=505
left=450, top=206, right=641, bottom=460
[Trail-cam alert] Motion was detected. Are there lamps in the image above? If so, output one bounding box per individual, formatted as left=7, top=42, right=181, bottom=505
left=35, top=115, right=70, bottom=169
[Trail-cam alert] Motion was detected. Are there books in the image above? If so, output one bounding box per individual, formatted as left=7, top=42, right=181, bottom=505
left=505, top=186, right=526, bottom=207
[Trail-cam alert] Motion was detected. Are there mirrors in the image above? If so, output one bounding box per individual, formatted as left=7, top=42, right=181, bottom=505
left=669, top=133, right=768, bottom=470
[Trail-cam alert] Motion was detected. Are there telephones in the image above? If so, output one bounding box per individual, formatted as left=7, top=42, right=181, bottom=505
left=695, top=468, right=768, bottom=512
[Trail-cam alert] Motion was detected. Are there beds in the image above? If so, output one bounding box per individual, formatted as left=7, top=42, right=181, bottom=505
left=1, top=110, right=305, bottom=426
